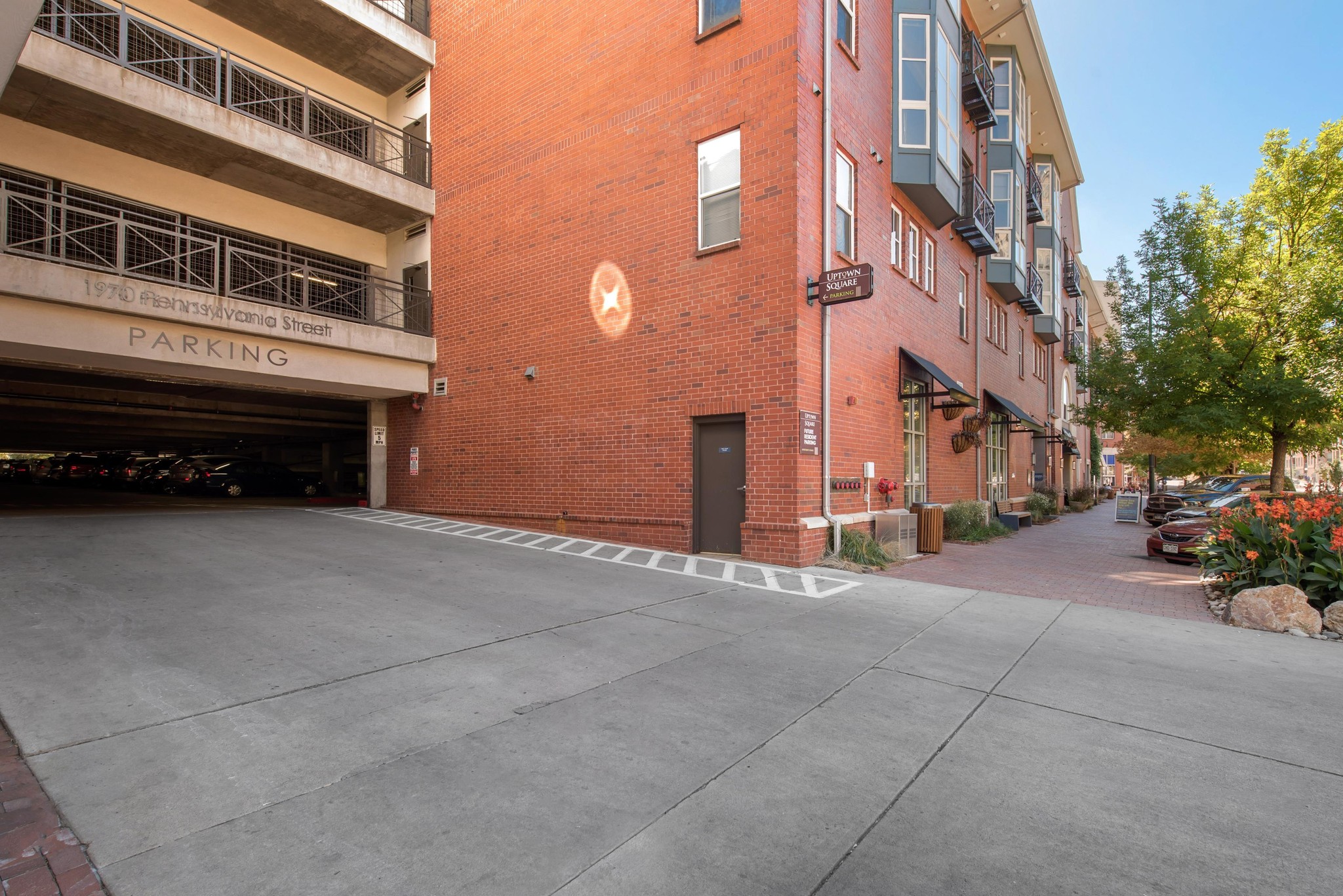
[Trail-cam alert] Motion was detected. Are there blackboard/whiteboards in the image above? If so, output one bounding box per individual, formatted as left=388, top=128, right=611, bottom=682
left=1116, top=496, right=1138, bottom=520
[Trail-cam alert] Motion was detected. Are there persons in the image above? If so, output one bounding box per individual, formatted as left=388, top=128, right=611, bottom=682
left=1101, top=481, right=1140, bottom=497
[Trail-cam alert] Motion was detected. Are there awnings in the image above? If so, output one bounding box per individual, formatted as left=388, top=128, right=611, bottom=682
left=1062, top=427, right=1081, bottom=455
left=983, top=388, right=1046, bottom=433
left=899, top=346, right=980, bottom=409
left=1094, top=450, right=1107, bottom=468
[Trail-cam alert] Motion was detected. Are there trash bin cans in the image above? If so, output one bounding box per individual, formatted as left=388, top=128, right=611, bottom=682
left=910, top=502, right=943, bottom=553
left=1107, top=489, right=1114, bottom=499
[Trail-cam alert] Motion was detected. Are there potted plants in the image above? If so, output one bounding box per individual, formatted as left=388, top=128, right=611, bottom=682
left=1082, top=481, right=1094, bottom=510
left=1067, top=479, right=1092, bottom=512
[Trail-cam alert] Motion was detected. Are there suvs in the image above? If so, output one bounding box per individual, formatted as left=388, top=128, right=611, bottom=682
left=1143, top=472, right=1297, bottom=524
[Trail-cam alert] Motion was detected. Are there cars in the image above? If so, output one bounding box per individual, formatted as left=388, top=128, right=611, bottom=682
left=1147, top=492, right=1316, bottom=568
left=0, top=451, right=331, bottom=501
